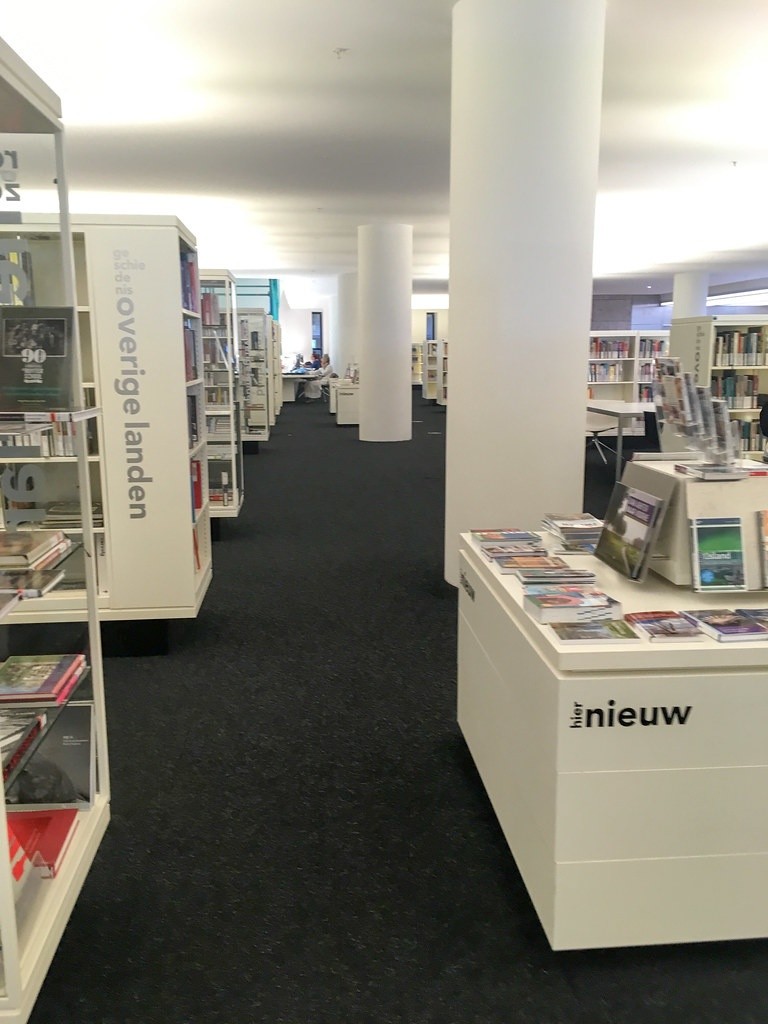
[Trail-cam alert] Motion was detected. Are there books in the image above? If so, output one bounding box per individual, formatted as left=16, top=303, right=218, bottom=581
left=411, top=325, right=768, bottom=646
left=0, top=252, right=266, bottom=905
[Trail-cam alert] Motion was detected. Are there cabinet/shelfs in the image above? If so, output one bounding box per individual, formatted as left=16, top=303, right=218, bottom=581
left=220, top=307, right=283, bottom=454
left=587, top=330, right=670, bottom=437
left=412, top=339, right=448, bottom=405
left=670, top=315, right=768, bottom=462
left=0, top=211, right=213, bottom=658
left=0, top=37, right=111, bottom=1024
left=198, top=269, right=244, bottom=542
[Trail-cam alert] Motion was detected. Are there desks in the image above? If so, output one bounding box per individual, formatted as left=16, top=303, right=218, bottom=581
left=282, top=375, right=318, bottom=402
left=588, top=402, right=654, bottom=482
left=454, top=520, right=768, bottom=953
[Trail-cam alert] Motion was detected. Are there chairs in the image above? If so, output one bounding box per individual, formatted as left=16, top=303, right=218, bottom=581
left=298, top=373, right=339, bottom=403
left=585, top=400, right=625, bottom=464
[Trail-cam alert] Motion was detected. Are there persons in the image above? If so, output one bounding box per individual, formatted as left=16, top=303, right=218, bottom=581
left=302, top=353, right=333, bottom=403
